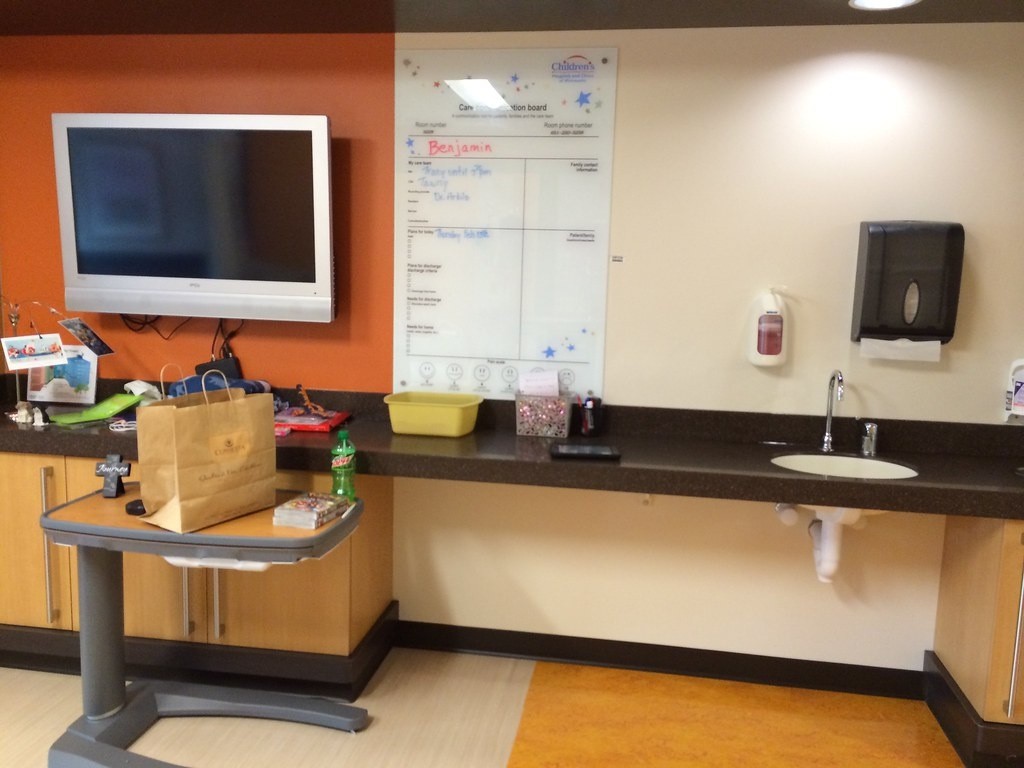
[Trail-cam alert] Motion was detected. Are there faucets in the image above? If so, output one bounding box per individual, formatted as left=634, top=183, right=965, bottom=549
left=823, top=369, right=846, bottom=452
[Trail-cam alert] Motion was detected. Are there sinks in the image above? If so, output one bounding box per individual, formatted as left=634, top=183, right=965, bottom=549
left=770, top=454, right=920, bottom=479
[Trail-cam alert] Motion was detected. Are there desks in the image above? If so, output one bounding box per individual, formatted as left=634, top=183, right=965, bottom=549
left=38, top=481, right=368, bottom=768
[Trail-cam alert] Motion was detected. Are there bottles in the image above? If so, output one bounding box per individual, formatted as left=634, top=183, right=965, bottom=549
left=331, top=431, right=356, bottom=502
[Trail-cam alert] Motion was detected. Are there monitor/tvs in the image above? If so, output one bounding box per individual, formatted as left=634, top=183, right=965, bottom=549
left=49, top=113, right=336, bottom=324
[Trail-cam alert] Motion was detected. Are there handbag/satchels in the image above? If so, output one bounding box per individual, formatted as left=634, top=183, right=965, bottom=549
left=136, top=363, right=276, bottom=533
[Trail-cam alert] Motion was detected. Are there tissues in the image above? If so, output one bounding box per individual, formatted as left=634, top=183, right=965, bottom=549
left=514, top=370, right=576, bottom=438
left=849, top=216, right=965, bottom=363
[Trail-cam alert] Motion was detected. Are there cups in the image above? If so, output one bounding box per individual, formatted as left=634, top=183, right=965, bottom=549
left=580, top=407, right=605, bottom=437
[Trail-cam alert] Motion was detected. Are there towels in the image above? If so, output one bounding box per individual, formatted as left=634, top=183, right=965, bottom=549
left=167, top=372, right=272, bottom=399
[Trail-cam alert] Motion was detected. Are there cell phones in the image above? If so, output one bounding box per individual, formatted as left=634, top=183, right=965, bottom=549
left=550, top=442, right=622, bottom=461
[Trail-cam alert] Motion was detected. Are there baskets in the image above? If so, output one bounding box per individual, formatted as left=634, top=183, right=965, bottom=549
left=515, top=390, right=575, bottom=439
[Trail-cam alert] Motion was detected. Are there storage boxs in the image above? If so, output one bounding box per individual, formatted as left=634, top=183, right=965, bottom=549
left=514, top=389, right=576, bottom=438
left=384, top=390, right=484, bottom=437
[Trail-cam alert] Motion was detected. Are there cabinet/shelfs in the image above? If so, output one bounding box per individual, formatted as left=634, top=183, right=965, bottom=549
left=65, top=456, right=402, bottom=704
left=0, top=451, right=73, bottom=675
